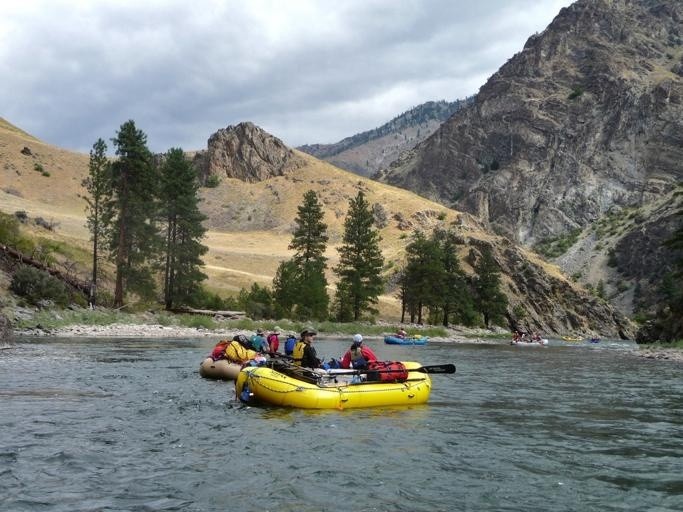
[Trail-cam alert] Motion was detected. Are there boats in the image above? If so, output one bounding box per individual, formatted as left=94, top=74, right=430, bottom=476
left=590, top=338, right=601, bottom=343
left=199, top=357, right=432, bottom=409
left=510, top=338, right=548, bottom=346
left=383, top=336, right=428, bottom=346
left=562, top=336, right=584, bottom=341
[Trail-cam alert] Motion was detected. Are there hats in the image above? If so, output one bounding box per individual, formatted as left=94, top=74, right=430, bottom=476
left=256, top=328, right=264, bottom=335
left=301, top=329, right=316, bottom=336
left=288, top=331, right=295, bottom=336
left=273, top=326, right=282, bottom=333
left=353, top=334, right=362, bottom=342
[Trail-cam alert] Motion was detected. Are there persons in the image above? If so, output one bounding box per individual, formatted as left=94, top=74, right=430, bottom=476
left=283, top=330, right=295, bottom=356
left=339, top=334, right=377, bottom=369
left=398, top=328, right=407, bottom=338
left=513, top=330, right=542, bottom=342
left=250, top=328, right=270, bottom=352
left=269, top=326, right=281, bottom=358
left=292, top=327, right=325, bottom=380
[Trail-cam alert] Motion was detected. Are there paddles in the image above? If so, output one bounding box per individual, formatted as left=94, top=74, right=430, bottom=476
left=327, top=364, right=456, bottom=374
